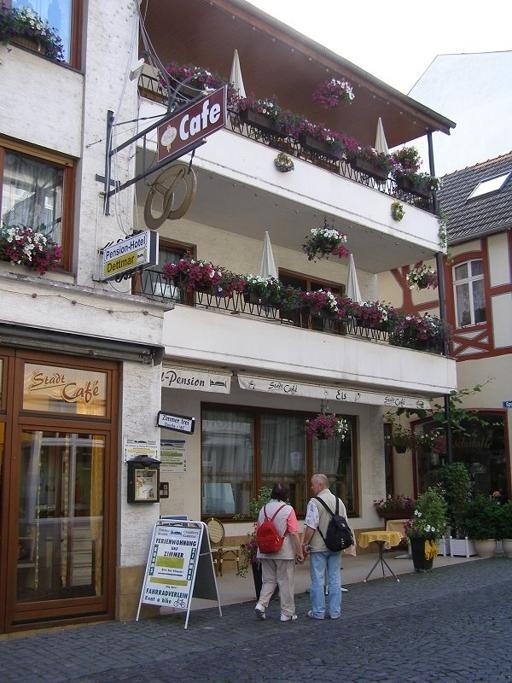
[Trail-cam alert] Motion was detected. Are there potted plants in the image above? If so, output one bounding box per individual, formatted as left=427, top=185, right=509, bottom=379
left=421, top=462, right=511, bottom=558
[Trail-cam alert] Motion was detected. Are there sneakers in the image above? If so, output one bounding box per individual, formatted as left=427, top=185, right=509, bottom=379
left=255, top=603, right=266, bottom=620
left=307, top=610, right=325, bottom=620
left=330, top=612, right=341, bottom=618
left=280, top=614, right=298, bottom=622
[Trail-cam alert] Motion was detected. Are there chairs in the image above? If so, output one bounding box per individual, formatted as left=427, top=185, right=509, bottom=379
left=204, top=516, right=243, bottom=577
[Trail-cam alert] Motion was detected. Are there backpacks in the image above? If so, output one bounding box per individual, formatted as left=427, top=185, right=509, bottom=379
left=257, top=503, right=290, bottom=554
left=314, top=495, right=354, bottom=552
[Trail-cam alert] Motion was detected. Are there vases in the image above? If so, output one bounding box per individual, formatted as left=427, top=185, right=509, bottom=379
left=251, top=562, right=279, bottom=600
left=394, top=445, right=406, bottom=453
left=9, top=31, right=48, bottom=55
left=409, top=536, right=435, bottom=569
left=373, top=494, right=417, bottom=512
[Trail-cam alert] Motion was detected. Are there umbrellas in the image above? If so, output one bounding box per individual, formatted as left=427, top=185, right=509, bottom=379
left=228, top=46, right=248, bottom=101
left=255, top=228, right=283, bottom=317
left=374, top=114, right=391, bottom=160
left=344, top=250, right=364, bottom=334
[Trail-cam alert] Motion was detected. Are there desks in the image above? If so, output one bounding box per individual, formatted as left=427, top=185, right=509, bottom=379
left=360, top=530, right=401, bottom=583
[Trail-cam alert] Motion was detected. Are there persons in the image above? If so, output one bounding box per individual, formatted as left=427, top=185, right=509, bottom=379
left=465, top=459, right=503, bottom=501
left=253, top=481, right=304, bottom=622
left=301, top=472, right=347, bottom=620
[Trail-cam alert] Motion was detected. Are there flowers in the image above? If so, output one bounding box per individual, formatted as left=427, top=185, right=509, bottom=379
left=301, top=225, right=350, bottom=262
left=403, top=485, right=448, bottom=540
left=1, top=0, right=66, bottom=62
left=0, top=221, right=63, bottom=275
left=233, top=484, right=291, bottom=578
left=384, top=423, right=440, bottom=448
left=163, top=249, right=454, bottom=345
left=305, top=413, right=348, bottom=442
left=156, top=61, right=444, bottom=192
